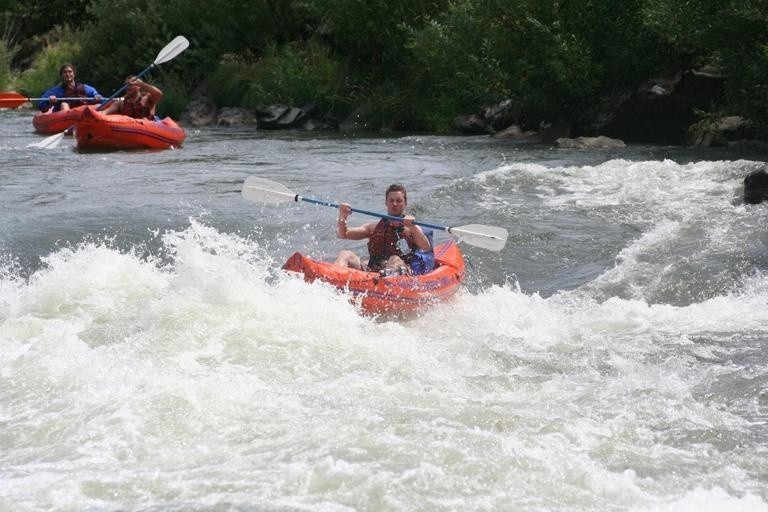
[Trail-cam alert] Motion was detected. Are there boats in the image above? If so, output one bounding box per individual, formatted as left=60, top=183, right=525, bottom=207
left=31, top=101, right=98, bottom=135
left=281, top=236, right=465, bottom=316
left=76, top=104, right=185, bottom=151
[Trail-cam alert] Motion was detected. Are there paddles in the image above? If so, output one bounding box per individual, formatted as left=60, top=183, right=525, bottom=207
left=0, top=91, right=125, bottom=108
left=241, top=176, right=508, bottom=252
left=36, top=35, right=190, bottom=149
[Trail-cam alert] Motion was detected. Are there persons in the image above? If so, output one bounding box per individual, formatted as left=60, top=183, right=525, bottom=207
left=97, top=76, right=164, bottom=123
left=333, top=184, right=432, bottom=279
left=36, top=64, right=105, bottom=114
